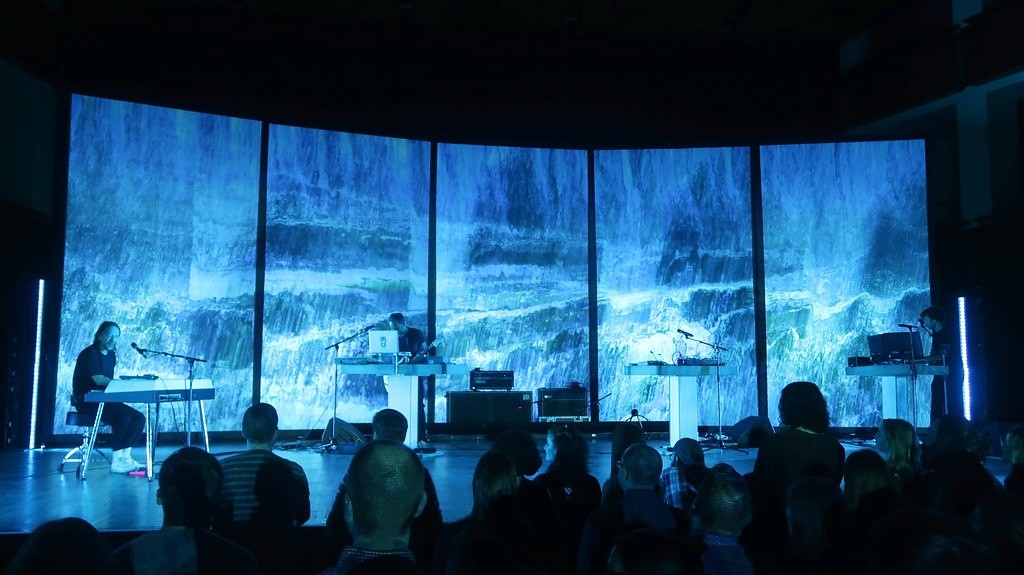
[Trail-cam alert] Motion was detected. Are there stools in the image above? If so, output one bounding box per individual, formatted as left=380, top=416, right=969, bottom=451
left=56, top=411, right=112, bottom=480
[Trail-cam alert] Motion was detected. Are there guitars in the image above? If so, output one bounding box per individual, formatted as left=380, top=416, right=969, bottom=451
left=383, top=337, right=445, bottom=394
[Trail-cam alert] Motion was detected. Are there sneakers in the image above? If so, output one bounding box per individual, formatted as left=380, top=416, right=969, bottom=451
left=125, top=458, right=144, bottom=470
left=111, top=460, right=136, bottom=473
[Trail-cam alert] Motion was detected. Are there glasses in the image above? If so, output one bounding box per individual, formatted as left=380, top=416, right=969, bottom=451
left=615, top=461, right=627, bottom=470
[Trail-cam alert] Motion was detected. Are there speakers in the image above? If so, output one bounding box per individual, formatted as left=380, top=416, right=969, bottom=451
left=726, top=416, right=775, bottom=448
left=538, top=387, right=587, bottom=417
left=321, top=417, right=365, bottom=445
left=446, top=391, right=533, bottom=427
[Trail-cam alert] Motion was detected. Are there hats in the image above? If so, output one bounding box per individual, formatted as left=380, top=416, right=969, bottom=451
left=667, top=439, right=702, bottom=465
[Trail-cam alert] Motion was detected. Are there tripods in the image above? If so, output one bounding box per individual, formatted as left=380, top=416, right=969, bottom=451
left=686, top=336, right=750, bottom=454
left=311, top=330, right=368, bottom=454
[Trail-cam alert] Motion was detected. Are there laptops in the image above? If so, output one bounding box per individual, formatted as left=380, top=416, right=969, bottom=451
left=867, top=332, right=925, bottom=363
left=368, top=330, right=400, bottom=354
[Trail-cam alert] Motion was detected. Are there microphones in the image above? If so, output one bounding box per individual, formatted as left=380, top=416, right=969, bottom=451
left=898, top=324, right=919, bottom=328
left=131, top=343, right=147, bottom=358
left=677, top=329, right=693, bottom=337
left=364, top=323, right=378, bottom=330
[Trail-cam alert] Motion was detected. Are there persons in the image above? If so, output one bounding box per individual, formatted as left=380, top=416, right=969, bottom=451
left=12, top=382, right=1024, bottom=574
left=72, top=321, right=147, bottom=474
left=921, top=306, right=964, bottom=425
left=389, top=313, right=431, bottom=448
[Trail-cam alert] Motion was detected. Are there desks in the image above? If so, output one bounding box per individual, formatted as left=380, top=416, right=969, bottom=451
left=846, top=364, right=950, bottom=444
left=624, top=364, right=738, bottom=449
left=340, top=364, right=469, bottom=458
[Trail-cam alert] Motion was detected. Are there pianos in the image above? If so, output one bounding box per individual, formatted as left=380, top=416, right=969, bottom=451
left=81, top=377, right=216, bottom=482
